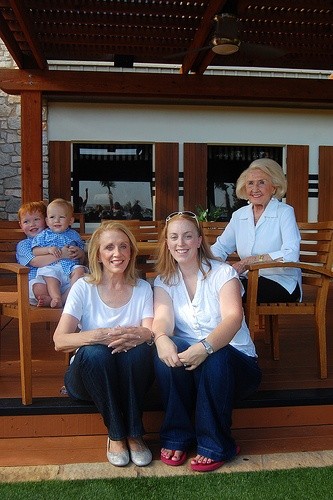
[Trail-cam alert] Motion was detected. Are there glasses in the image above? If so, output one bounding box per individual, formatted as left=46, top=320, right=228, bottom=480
left=165, top=211, right=200, bottom=229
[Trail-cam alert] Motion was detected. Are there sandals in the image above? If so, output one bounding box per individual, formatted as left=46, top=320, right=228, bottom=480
left=190, top=445, right=241, bottom=472
left=160, top=447, right=187, bottom=466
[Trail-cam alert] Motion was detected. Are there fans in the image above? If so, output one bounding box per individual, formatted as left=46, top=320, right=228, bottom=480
left=155, top=8, right=305, bottom=70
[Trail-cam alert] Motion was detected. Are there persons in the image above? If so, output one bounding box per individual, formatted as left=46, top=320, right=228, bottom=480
left=152, top=211, right=259, bottom=470
left=208, top=160, right=302, bottom=304
left=16, top=199, right=90, bottom=307
left=53, top=220, right=155, bottom=467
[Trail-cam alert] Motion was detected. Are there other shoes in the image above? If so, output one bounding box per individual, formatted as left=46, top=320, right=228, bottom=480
left=107, top=436, right=130, bottom=468
left=129, top=436, right=153, bottom=467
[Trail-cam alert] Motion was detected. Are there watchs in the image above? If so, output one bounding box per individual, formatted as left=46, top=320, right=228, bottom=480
left=200, top=339, right=214, bottom=355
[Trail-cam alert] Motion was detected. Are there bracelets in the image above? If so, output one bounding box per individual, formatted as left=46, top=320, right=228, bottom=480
left=147, top=331, right=154, bottom=346
left=155, top=333, right=170, bottom=343
left=259, top=255, right=263, bottom=263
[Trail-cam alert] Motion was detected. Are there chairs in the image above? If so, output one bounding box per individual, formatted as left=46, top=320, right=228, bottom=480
left=0, top=210, right=333, bottom=407
